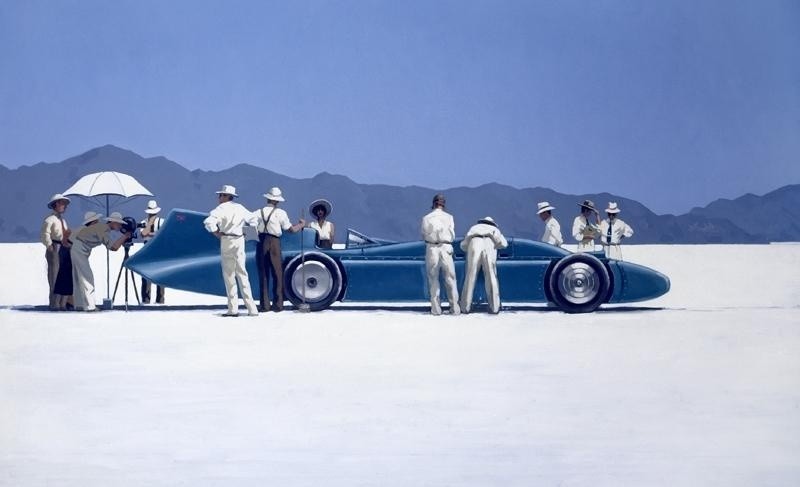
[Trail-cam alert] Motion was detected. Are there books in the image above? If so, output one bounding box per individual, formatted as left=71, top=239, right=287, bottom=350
left=242, top=226, right=260, bottom=241
left=581, top=225, right=597, bottom=242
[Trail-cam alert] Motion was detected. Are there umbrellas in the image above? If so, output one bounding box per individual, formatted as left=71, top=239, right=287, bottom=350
left=62, top=171, right=154, bottom=299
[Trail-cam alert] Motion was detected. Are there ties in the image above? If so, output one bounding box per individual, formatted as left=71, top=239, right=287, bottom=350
left=607, top=222, right=611, bottom=243
left=60, top=218, right=71, bottom=248
left=586, top=218, right=589, bottom=225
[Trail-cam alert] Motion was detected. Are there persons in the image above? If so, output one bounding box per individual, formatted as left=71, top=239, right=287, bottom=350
left=572, top=200, right=601, bottom=252
left=457, top=216, right=509, bottom=314
left=536, top=201, right=564, bottom=247
left=139, top=200, right=165, bottom=304
left=204, top=185, right=258, bottom=315
left=420, top=194, right=461, bottom=315
left=253, top=188, right=305, bottom=313
left=41, top=192, right=130, bottom=311
left=307, top=199, right=335, bottom=248
left=599, top=201, right=634, bottom=261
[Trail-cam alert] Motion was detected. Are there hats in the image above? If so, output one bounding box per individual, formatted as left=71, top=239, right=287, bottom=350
left=82, top=211, right=103, bottom=225
left=576, top=200, right=596, bottom=212
left=214, top=186, right=239, bottom=197
left=478, top=217, right=498, bottom=227
left=48, top=194, right=71, bottom=209
left=309, top=200, right=333, bottom=220
left=536, top=202, right=555, bottom=215
left=144, top=200, right=162, bottom=215
left=263, top=188, right=285, bottom=202
left=605, top=202, right=621, bottom=214
left=105, top=212, right=128, bottom=224
